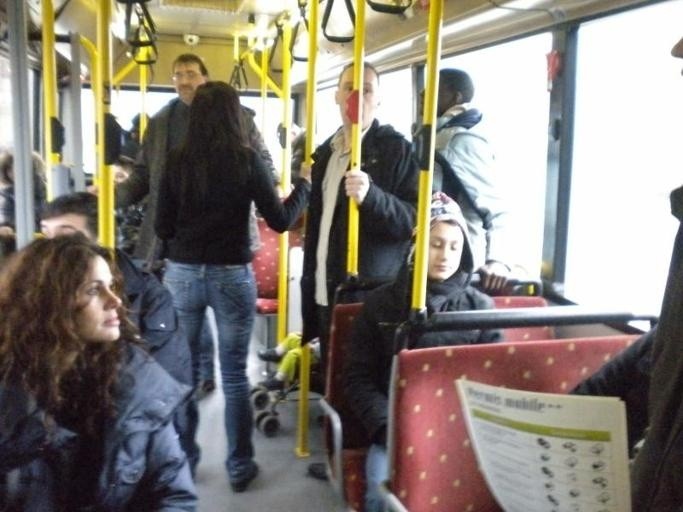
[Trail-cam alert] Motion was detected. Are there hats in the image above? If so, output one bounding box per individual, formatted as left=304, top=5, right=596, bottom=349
left=406, top=191, right=474, bottom=274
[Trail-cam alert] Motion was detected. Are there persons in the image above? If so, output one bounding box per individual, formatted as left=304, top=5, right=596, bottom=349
left=569, top=185, right=683, bottom=512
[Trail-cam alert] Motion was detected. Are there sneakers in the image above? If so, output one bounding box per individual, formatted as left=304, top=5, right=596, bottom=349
left=257, top=348, right=281, bottom=364
left=257, top=377, right=285, bottom=391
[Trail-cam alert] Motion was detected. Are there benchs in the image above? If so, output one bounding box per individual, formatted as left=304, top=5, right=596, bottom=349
left=253, top=216, right=650, bottom=511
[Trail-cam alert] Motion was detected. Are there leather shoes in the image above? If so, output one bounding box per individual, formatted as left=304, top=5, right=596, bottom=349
left=231, top=464, right=258, bottom=492
left=308, top=463, right=326, bottom=479
